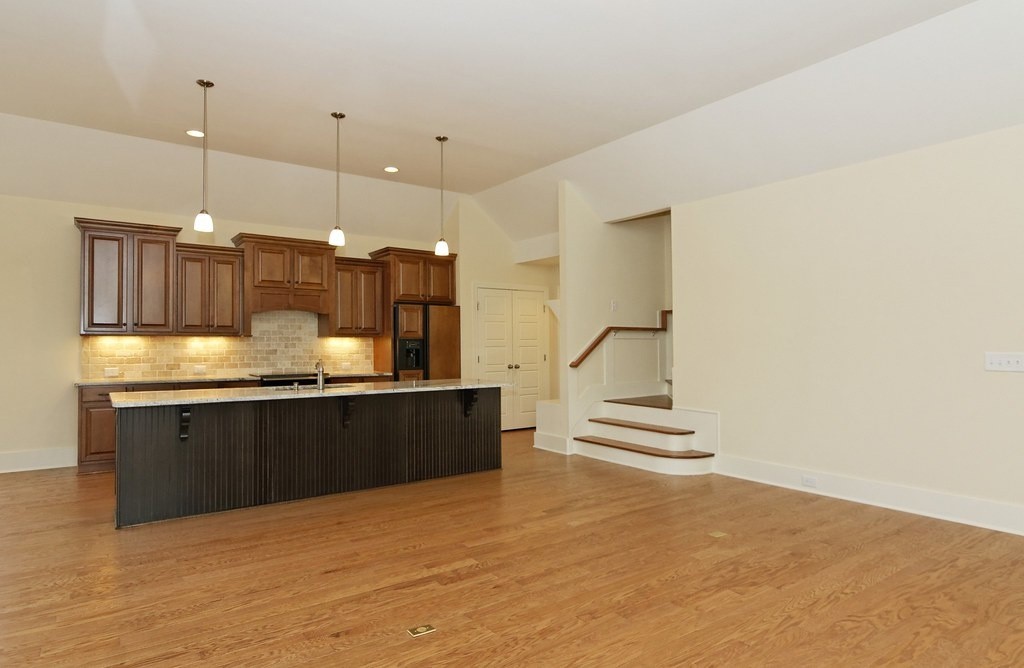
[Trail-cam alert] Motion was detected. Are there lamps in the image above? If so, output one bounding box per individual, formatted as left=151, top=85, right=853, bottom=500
left=434, top=136, right=449, bottom=257
left=194, top=79, right=214, bottom=234
left=328, top=112, right=347, bottom=248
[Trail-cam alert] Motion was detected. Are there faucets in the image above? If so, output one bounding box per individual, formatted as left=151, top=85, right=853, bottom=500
left=314, top=360, right=325, bottom=391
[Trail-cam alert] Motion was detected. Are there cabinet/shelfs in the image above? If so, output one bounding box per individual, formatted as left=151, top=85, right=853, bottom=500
left=231, top=232, right=338, bottom=337
left=74, top=217, right=184, bottom=336
left=176, top=243, right=244, bottom=337
left=317, top=256, right=391, bottom=338
left=78, top=379, right=261, bottom=473
left=368, top=246, right=458, bottom=304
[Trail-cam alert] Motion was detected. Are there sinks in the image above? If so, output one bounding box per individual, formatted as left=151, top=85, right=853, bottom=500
left=271, top=384, right=355, bottom=391
